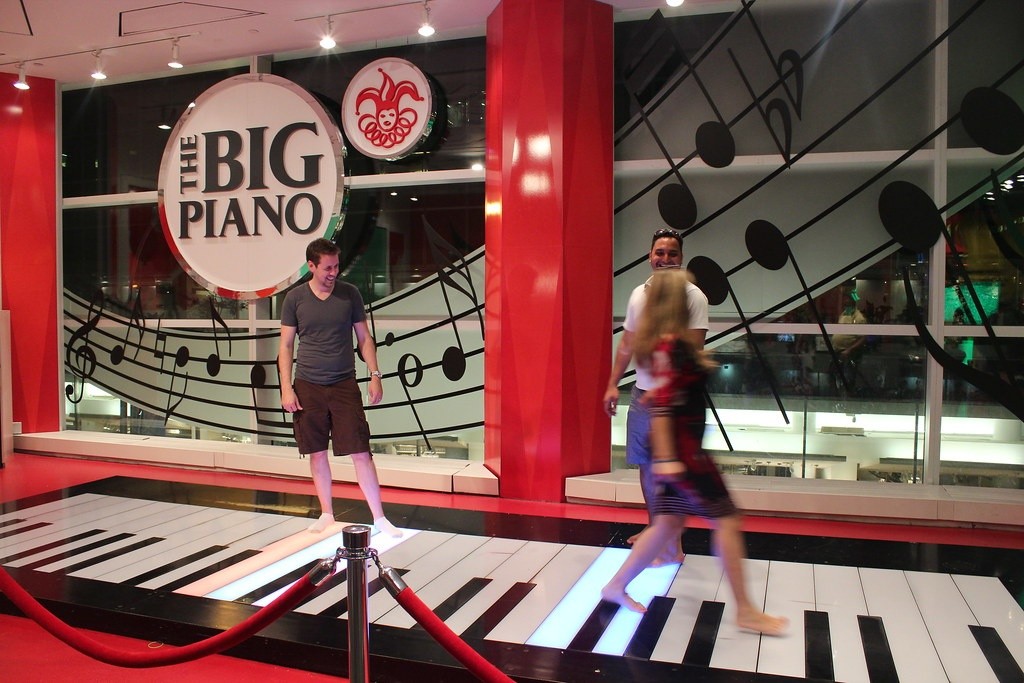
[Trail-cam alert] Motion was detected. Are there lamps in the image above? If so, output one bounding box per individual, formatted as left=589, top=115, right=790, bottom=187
left=13, top=34, right=183, bottom=91
left=318, top=0, right=435, bottom=50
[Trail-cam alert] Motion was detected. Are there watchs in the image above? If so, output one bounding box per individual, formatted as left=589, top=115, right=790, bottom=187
left=370, top=371, right=382, bottom=378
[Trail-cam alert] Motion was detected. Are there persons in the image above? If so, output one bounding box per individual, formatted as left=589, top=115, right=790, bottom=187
left=602, top=229, right=709, bottom=570
left=151, top=281, right=179, bottom=319
left=602, top=270, right=792, bottom=635
left=832, top=295, right=866, bottom=398
left=278, top=238, right=402, bottom=538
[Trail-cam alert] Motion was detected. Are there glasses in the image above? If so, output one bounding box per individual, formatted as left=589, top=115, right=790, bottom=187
left=652, top=229, right=683, bottom=246
